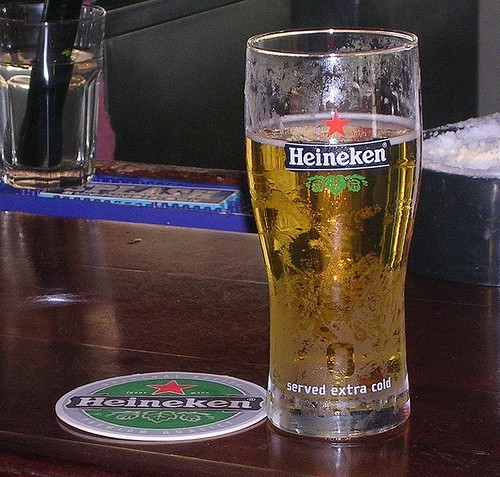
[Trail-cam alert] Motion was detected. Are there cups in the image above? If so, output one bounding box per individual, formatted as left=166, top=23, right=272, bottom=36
left=1, top=2, right=107, bottom=191
left=244, top=29, right=422, bottom=440
